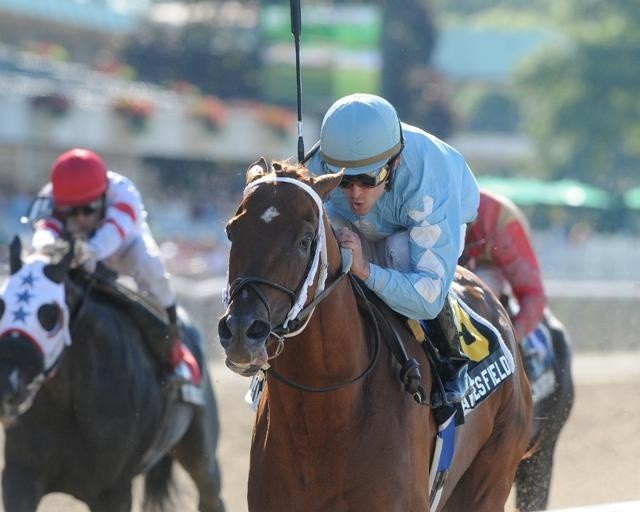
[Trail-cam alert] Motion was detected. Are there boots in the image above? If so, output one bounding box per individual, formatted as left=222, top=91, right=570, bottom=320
left=157, top=303, right=193, bottom=390
left=422, top=292, right=474, bottom=409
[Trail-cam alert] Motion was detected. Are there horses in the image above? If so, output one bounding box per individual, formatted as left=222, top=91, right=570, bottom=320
left=0, top=234, right=223, bottom=512
left=216, top=156, right=575, bottom=512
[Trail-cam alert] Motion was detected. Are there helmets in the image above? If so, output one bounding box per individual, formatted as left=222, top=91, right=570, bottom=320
left=52, top=149, right=108, bottom=207
left=320, top=93, right=402, bottom=176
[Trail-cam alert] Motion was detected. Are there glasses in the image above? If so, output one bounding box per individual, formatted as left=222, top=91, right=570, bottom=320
left=58, top=202, right=100, bottom=218
left=329, top=147, right=404, bottom=189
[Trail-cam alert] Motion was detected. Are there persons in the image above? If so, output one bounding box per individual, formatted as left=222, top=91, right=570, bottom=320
left=459, top=188, right=548, bottom=347
left=240, top=90, right=479, bottom=416
left=29, top=148, right=196, bottom=381
